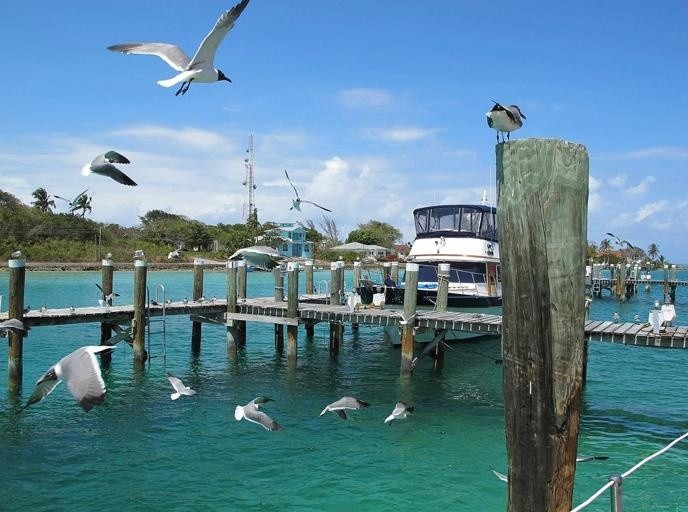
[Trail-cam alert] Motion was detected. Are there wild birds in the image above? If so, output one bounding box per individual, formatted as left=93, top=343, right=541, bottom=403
left=285, top=169, right=332, bottom=213
left=612, top=312, right=621, bottom=324
left=151, top=296, right=217, bottom=306
left=234, top=397, right=283, bottom=432
left=576, top=456, right=610, bottom=462
left=14, top=345, right=119, bottom=415
left=95, top=283, right=121, bottom=307
left=490, top=470, right=508, bottom=483
left=81, top=151, right=138, bottom=186
left=606, top=233, right=634, bottom=249
left=389, top=309, right=424, bottom=324
left=167, top=371, right=197, bottom=400
left=320, top=396, right=372, bottom=420
left=0, top=318, right=32, bottom=338
left=53, top=189, right=88, bottom=206
left=106, top=0, right=250, bottom=96
left=633, top=314, right=641, bottom=325
left=384, top=401, right=416, bottom=426
left=483, top=99, right=526, bottom=143
left=23, top=306, right=75, bottom=317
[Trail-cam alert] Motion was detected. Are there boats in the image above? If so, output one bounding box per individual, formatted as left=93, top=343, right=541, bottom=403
left=349, top=188, right=504, bottom=345
